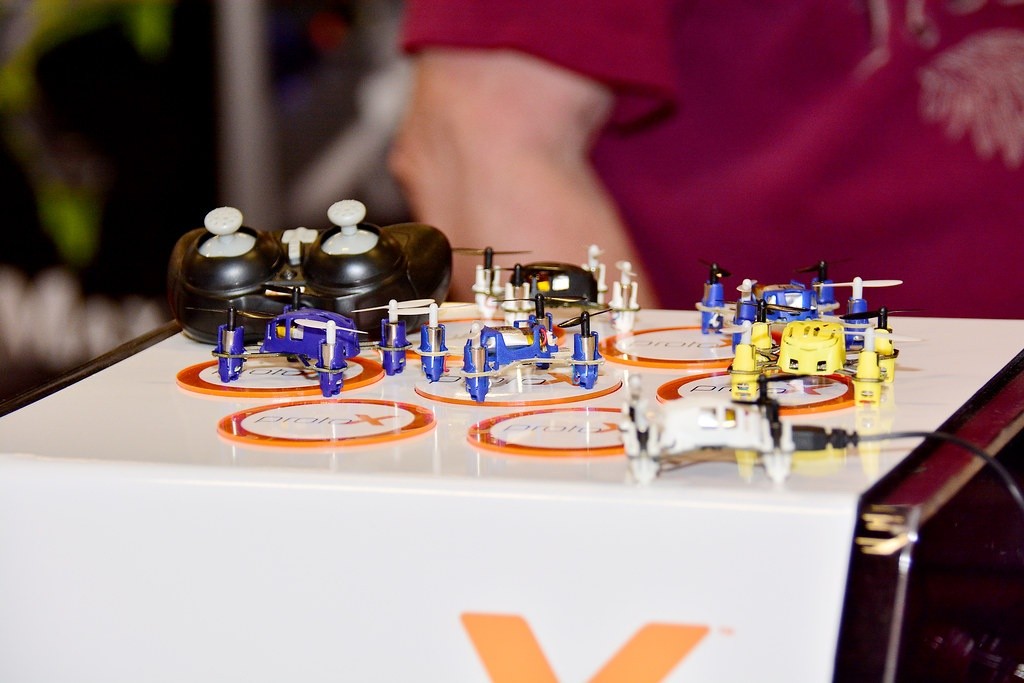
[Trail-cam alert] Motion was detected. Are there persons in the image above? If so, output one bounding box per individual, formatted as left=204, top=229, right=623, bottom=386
left=388, top=0, right=1023, bottom=319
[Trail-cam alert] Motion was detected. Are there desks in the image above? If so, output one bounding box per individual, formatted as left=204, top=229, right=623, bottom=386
left=0, top=307, right=1024, bottom=683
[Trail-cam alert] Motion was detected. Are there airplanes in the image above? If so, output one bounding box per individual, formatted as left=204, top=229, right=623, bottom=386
left=183, top=283, right=433, bottom=397
left=409, top=247, right=924, bottom=488
